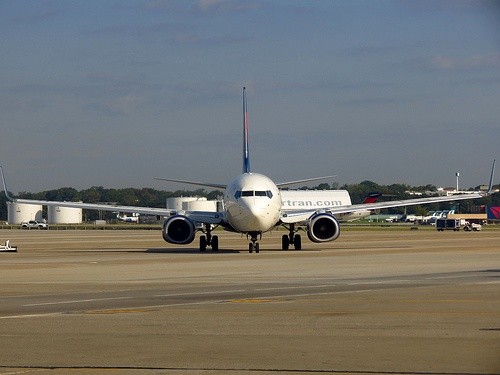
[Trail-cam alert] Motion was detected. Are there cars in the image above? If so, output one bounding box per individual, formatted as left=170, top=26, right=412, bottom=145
left=410, top=225, right=418, bottom=231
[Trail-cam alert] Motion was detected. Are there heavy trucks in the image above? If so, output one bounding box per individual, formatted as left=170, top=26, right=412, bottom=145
left=434, top=217, right=482, bottom=232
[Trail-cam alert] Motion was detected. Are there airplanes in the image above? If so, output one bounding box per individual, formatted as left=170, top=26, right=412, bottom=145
left=0, top=85, right=498, bottom=255
left=113, top=210, right=140, bottom=224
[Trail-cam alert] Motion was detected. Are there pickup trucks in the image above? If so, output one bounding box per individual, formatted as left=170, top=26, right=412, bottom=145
left=21, top=218, right=49, bottom=231
